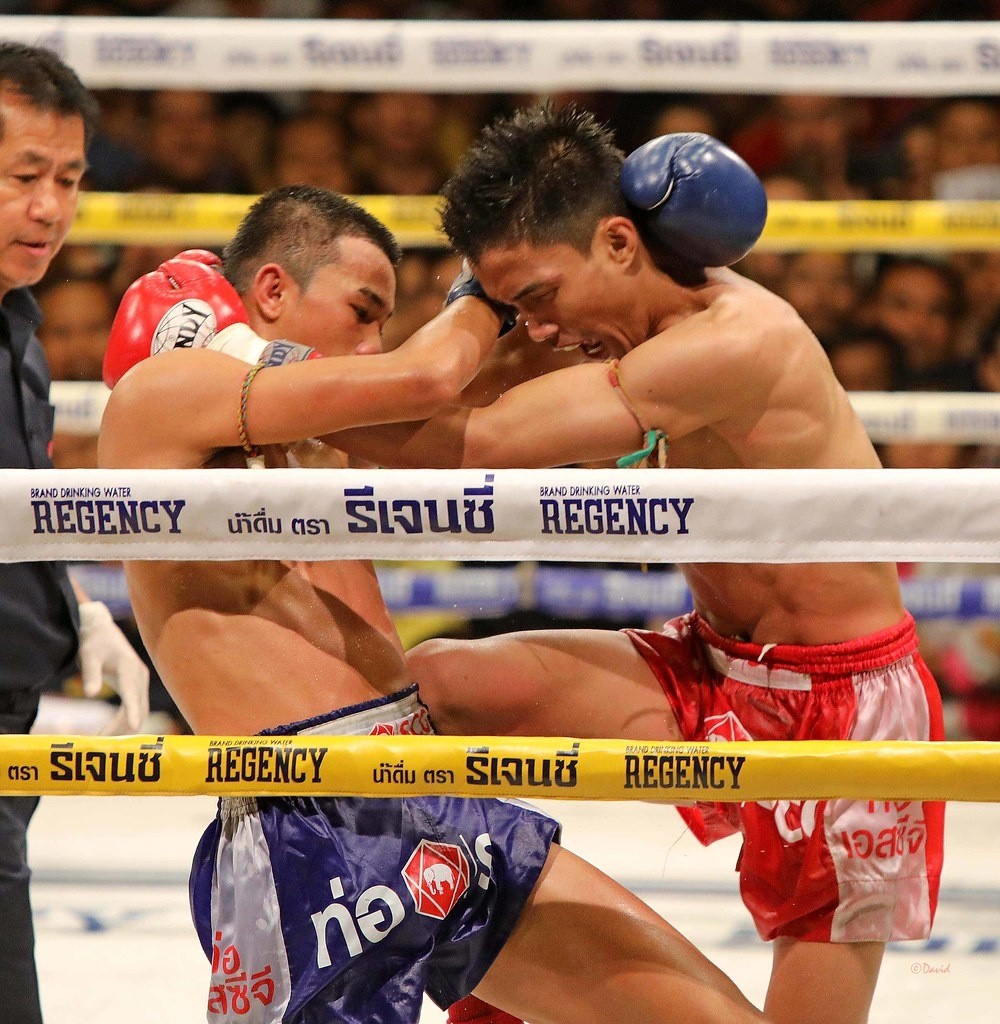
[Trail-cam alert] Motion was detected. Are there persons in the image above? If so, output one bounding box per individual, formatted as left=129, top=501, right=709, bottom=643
left=0, top=40, right=100, bottom=1024
left=103, top=100, right=947, bottom=1024
left=0, top=0, right=1000, bottom=467
left=97, top=132, right=768, bottom=1024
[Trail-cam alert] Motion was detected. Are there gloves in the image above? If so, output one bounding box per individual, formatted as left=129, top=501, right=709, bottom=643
left=443, top=254, right=521, bottom=340
left=173, top=249, right=222, bottom=277
left=621, top=133, right=767, bottom=269
left=75, top=599, right=150, bottom=732
left=102, top=258, right=320, bottom=390
